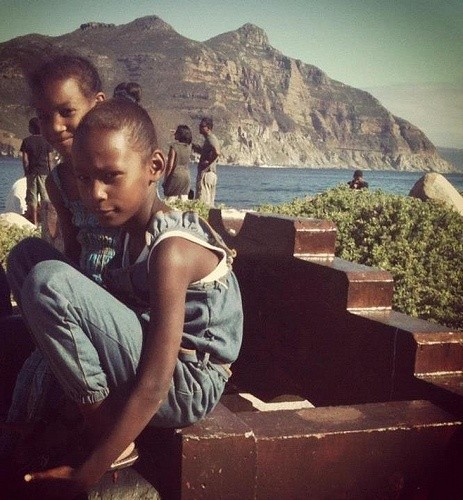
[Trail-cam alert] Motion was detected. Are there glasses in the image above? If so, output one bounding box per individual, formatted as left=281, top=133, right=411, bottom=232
left=200, top=125, right=208, bottom=129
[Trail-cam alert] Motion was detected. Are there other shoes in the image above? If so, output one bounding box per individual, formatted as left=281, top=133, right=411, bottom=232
left=70, top=439, right=139, bottom=472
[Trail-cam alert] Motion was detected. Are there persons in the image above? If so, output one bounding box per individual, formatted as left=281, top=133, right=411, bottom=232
left=348, top=169, right=368, bottom=191
left=0, top=50, right=244, bottom=500
